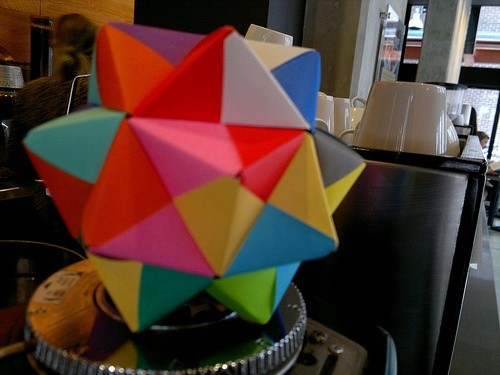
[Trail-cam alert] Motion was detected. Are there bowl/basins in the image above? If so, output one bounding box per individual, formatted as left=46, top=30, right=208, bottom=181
left=245, top=24, right=294, bottom=46
left=314, top=80, right=460, bottom=157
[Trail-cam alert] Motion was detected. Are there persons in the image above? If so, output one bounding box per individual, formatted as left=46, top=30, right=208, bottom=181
left=476, top=130, right=499, bottom=232
left=4, top=13, right=99, bottom=287
